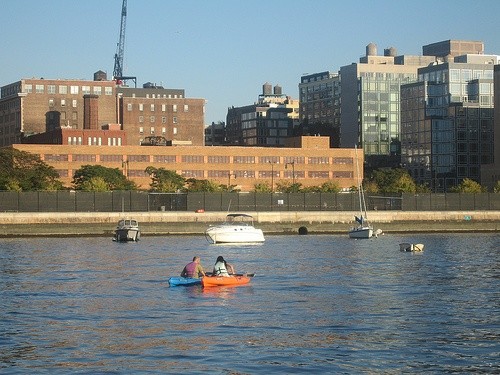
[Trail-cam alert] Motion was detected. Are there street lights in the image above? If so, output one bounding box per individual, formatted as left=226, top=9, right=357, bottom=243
left=284, top=162, right=295, bottom=184
left=269, top=160, right=276, bottom=192
left=228, top=173, right=236, bottom=186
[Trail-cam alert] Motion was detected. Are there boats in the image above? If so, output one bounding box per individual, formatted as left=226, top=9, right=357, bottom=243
left=168, top=275, right=202, bottom=286
left=201, top=274, right=251, bottom=292
left=111, top=218, right=140, bottom=241
left=206, top=212, right=267, bottom=245
left=349, top=144, right=372, bottom=238
left=398, top=242, right=424, bottom=253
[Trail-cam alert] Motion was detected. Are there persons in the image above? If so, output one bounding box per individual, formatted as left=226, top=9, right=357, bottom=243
left=180, top=256, right=205, bottom=279
left=212, top=256, right=236, bottom=277
